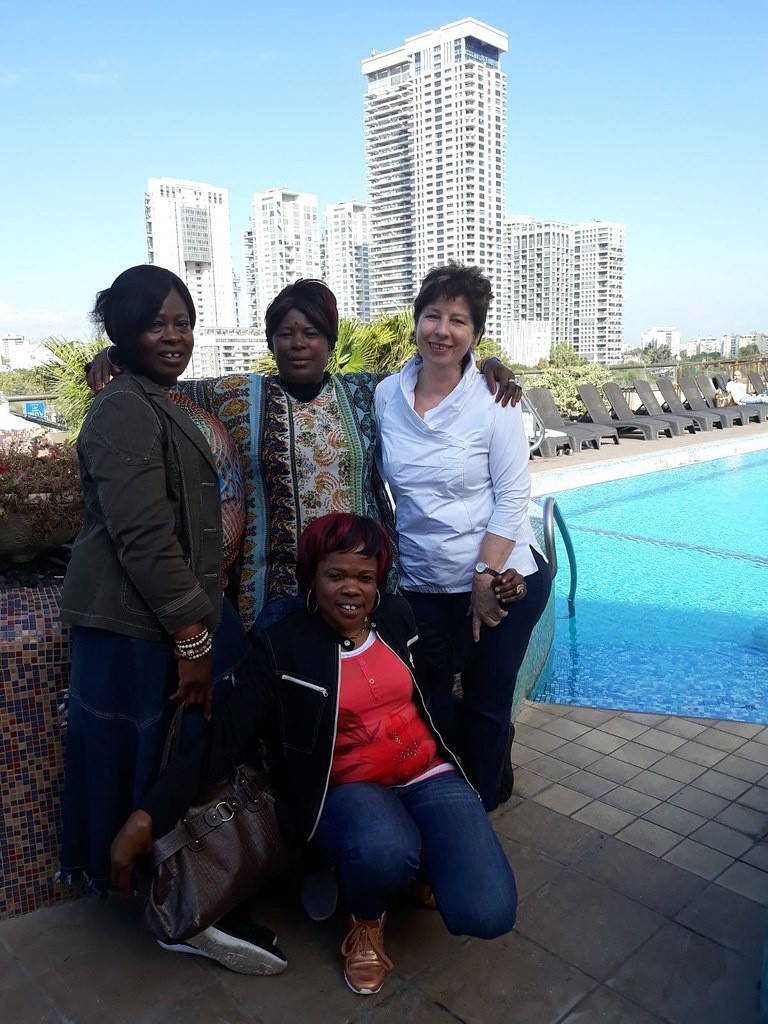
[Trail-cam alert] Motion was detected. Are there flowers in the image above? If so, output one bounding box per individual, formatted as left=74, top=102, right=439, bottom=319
left=0, top=446, right=83, bottom=536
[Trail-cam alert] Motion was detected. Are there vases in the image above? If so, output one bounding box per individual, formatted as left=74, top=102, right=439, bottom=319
left=0, top=487, right=83, bottom=564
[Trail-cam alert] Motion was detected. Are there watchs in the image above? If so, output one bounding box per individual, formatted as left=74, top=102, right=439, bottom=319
left=476, top=561, right=498, bottom=578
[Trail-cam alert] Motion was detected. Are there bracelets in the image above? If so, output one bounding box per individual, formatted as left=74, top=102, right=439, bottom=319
left=174, top=628, right=212, bottom=659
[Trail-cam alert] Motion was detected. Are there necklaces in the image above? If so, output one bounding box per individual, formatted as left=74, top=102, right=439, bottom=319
left=350, top=616, right=368, bottom=639
left=276, top=379, right=322, bottom=405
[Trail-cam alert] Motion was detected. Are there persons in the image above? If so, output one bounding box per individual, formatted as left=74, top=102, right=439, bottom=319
left=81, top=275, right=525, bottom=644
left=56, top=265, right=290, bottom=975
left=373, top=260, right=553, bottom=809
left=111, top=514, right=523, bottom=992
left=725, top=370, right=768, bottom=406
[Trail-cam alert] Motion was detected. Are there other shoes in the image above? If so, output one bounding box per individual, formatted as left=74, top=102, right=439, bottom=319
left=300, top=855, right=339, bottom=921
left=498, top=721, right=516, bottom=804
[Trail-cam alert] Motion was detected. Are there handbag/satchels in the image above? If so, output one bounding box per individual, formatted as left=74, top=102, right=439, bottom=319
left=141, top=697, right=291, bottom=945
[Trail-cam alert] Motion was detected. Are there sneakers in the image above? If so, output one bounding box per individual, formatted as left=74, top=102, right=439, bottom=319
left=340, top=909, right=394, bottom=995
left=411, top=876, right=439, bottom=910
left=157, top=905, right=288, bottom=976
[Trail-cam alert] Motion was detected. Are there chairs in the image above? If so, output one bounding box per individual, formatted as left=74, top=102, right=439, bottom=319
left=696, top=375, right=766, bottom=422
left=657, top=379, right=733, bottom=428
left=633, top=380, right=695, bottom=436
left=679, top=377, right=749, bottom=425
left=577, top=385, right=674, bottom=440
left=715, top=374, right=768, bottom=419
left=528, top=389, right=600, bottom=452
left=602, top=382, right=684, bottom=436
left=748, top=371, right=768, bottom=396
left=566, top=422, right=619, bottom=444
left=532, top=429, right=574, bottom=458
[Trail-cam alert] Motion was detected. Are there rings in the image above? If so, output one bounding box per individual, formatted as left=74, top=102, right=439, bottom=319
left=515, top=584, right=525, bottom=595
left=507, top=378, right=515, bottom=382
